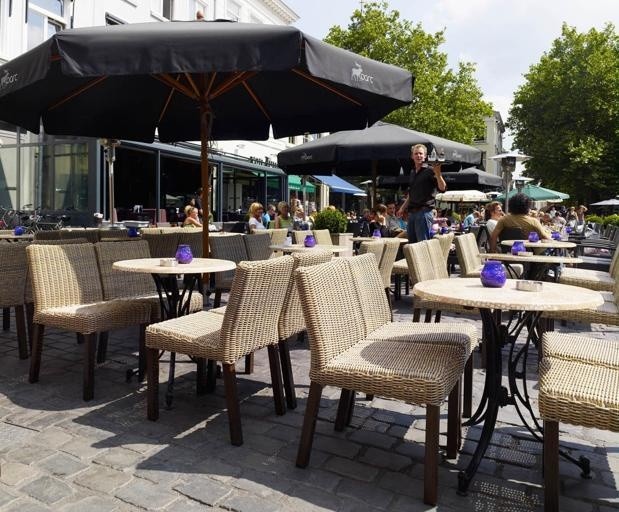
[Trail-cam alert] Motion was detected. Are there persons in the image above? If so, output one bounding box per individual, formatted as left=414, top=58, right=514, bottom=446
left=358, top=198, right=410, bottom=238
left=183, top=197, right=213, bottom=228
left=308, top=208, right=318, bottom=223
left=482, top=195, right=589, bottom=281
left=432, top=209, right=481, bottom=234
left=396, top=142, right=448, bottom=244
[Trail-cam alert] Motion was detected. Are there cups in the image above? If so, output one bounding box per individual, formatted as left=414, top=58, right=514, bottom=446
left=427, top=155, right=445, bottom=161
left=284, top=236, right=292, bottom=246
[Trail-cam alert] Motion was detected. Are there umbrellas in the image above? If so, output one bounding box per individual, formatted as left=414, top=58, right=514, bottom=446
left=433, top=167, right=619, bottom=207
left=0, top=10, right=416, bottom=313
left=279, top=125, right=483, bottom=214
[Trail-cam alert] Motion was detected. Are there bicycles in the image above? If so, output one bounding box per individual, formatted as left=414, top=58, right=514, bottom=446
left=0, top=202, right=73, bottom=236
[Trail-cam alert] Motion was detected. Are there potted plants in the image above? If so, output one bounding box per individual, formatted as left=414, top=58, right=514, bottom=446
left=313, top=209, right=354, bottom=256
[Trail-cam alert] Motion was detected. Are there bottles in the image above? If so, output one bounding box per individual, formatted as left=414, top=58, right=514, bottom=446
left=511, top=241, right=526, bottom=256
left=174, top=243, right=193, bottom=264
left=373, top=229, right=381, bottom=238
left=14, top=224, right=24, bottom=236
left=428, top=224, right=467, bottom=236
left=305, top=234, right=316, bottom=247
left=480, top=260, right=507, bottom=287
left=431, top=147, right=445, bottom=161
left=128, top=225, right=138, bottom=238
left=528, top=226, right=572, bottom=242
